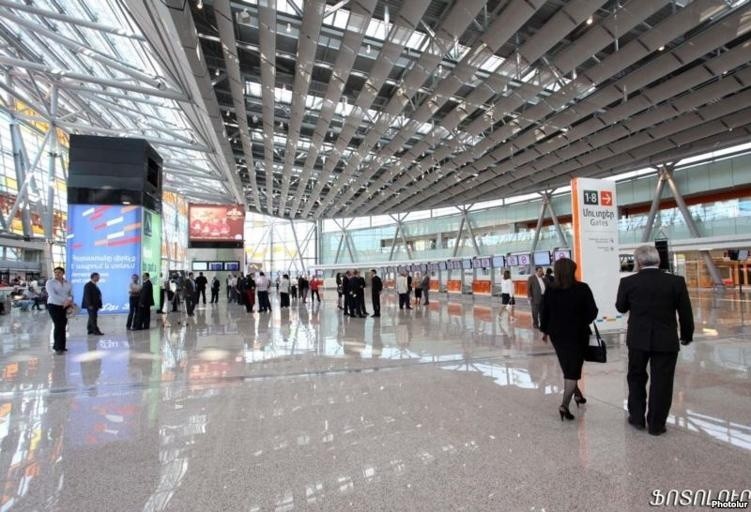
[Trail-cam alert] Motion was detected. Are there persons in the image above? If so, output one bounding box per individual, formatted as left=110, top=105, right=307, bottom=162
left=526, top=266, right=549, bottom=330
left=545, top=268, right=554, bottom=281
left=616, top=245, right=694, bottom=435
left=539, top=258, right=598, bottom=420
left=498, top=270, right=518, bottom=321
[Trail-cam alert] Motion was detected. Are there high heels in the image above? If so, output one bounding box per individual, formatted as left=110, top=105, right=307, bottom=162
left=574, top=394, right=586, bottom=407
left=559, top=405, right=575, bottom=421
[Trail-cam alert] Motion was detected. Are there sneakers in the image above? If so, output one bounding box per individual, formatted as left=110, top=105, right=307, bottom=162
left=336, top=300, right=430, bottom=318
left=628, top=415, right=666, bottom=436
left=156, top=300, right=288, bottom=316
left=494, top=315, right=540, bottom=329
left=54, top=324, right=150, bottom=355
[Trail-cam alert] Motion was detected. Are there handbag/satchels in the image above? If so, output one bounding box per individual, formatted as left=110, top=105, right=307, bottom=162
left=584, top=321, right=607, bottom=363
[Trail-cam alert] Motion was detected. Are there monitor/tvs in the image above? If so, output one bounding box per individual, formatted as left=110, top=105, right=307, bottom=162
left=191, top=261, right=208, bottom=271
left=380, top=248, right=572, bottom=273
left=223, top=260, right=240, bottom=271
left=208, top=261, right=223, bottom=271
